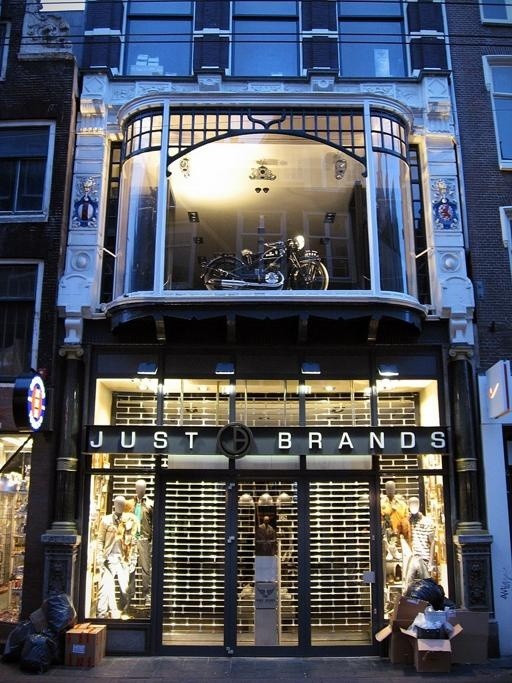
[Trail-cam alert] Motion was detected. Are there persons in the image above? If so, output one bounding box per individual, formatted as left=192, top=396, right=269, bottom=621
left=123, top=479, right=154, bottom=607
left=380, top=480, right=408, bottom=562
left=401, top=496, right=437, bottom=596
left=97, top=496, right=138, bottom=620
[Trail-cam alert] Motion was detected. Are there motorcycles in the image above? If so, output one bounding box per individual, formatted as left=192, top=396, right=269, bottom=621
left=200, top=235, right=330, bottom=290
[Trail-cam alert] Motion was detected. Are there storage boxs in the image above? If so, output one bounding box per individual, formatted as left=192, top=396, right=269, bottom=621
left=374, top=597, right=489, bottom=673
left=66, top=624, right=107, bottom=667
